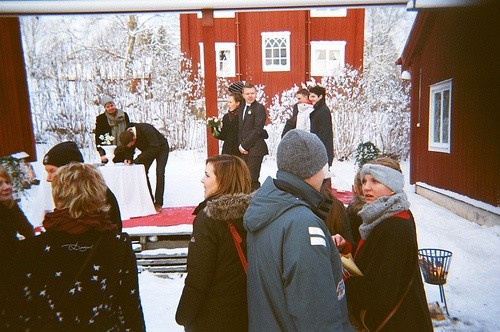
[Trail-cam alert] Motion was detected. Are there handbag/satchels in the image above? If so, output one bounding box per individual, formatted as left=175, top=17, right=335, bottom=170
left=349, top=310, right=375, bottom=332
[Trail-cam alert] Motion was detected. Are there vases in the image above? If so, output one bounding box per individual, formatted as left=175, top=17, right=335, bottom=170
left=101, top=145, right=118, bottom=167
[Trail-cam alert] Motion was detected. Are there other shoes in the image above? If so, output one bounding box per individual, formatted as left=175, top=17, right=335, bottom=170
left=154, top=203, right=162, bottom=212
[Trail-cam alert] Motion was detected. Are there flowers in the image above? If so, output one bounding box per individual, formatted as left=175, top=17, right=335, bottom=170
left=205, top=116, right=222, bottom=138
left=98, top=132, right=115, bottom=145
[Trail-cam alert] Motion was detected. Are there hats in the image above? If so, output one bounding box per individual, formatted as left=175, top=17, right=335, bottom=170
left=102, top=94, right=114, bottom=108
left=43, top=141, right=84, bottom=168
left=277, top=129, right=328, bottom=179
left=228, top=81, right=243, bottom=94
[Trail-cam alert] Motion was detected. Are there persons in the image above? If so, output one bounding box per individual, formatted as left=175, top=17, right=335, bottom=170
left=0, top=161, right=146, bottom=332
left=243, top=129, right=355, bottom=332
left=281, top=85, right=335, bottom=168
left=119, top=122, right=169, bottom=212
left=331, top=158, right=434, bottom=332
left=43, top=141, right=123, bottom=233
left=211, top=82, right=269, bottom=192
left=313, top=181, right=355, bottom=248
left=0, top=165, right=35, bottom=265
left=176, top=154, right=255, bottom=332
left=347, top=167, right=367, bottom=241
left=95, top=95, right=131, bottom=163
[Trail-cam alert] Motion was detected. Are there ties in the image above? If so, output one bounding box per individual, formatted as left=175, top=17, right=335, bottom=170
left=244, top=106, right=249, bottom=125
